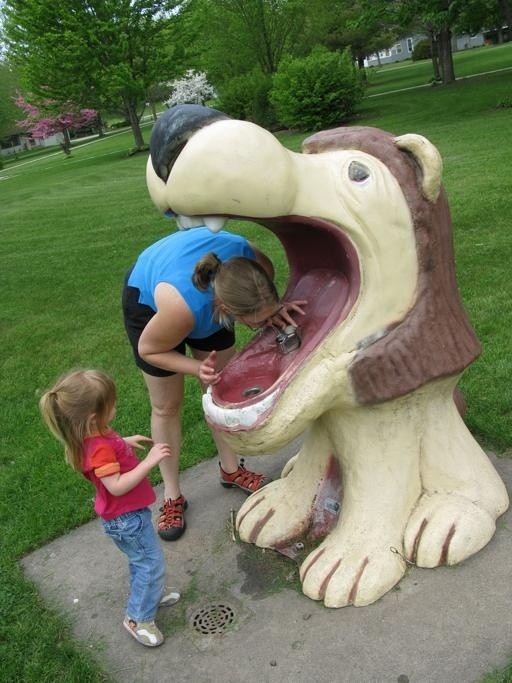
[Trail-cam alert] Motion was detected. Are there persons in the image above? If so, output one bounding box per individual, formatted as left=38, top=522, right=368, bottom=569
left=39, top=370, right=182, bottom=648
left=121, top=226, right=309, bottom=542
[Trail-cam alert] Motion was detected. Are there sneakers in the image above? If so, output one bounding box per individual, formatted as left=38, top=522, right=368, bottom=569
left=156, top=495, right=188, bottom=540
left=123, top=613, right=164, bottom=647
left=158, top=591, right=180, bottom=608
left=217, top=461, right=273, bottom=496
